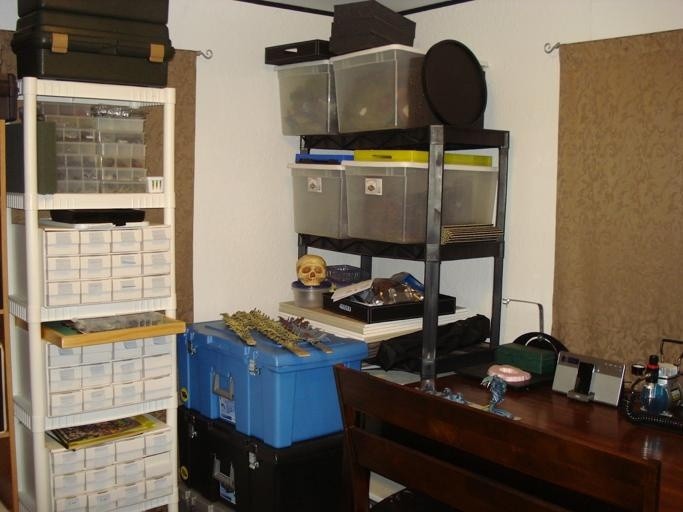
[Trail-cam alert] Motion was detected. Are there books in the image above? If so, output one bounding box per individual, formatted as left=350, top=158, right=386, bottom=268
left=47, top=418, right=163, bottom=450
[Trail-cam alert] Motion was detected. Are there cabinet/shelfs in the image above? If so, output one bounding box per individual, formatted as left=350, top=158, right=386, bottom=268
left=298, top=124, right=511, bottom=381
left=0, top=118, right=20, bottom=512
left=6, top=78, right=178, bottom=511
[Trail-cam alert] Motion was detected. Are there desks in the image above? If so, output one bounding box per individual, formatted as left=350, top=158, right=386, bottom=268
left=394, top=367, right=683, bottom=511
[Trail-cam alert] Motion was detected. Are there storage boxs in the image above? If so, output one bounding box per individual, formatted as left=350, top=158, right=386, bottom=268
left=274, top=60, right=337, bottom=134
left=287, top=160, right=346, bottom=237
left=343, top=162, right=499, bottom=245
left=177, top=407, right=345, bottom=512
left=11, top=10, right=174, bottom=89
left=331, top=43, right=487, bottom=132
left=176, top=319, right=368, bottom=449
left=17, top=0, right=169, bottom=25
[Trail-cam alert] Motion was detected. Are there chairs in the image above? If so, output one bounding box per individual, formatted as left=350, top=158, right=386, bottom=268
left=331, top=362, right=660, bottom=512
